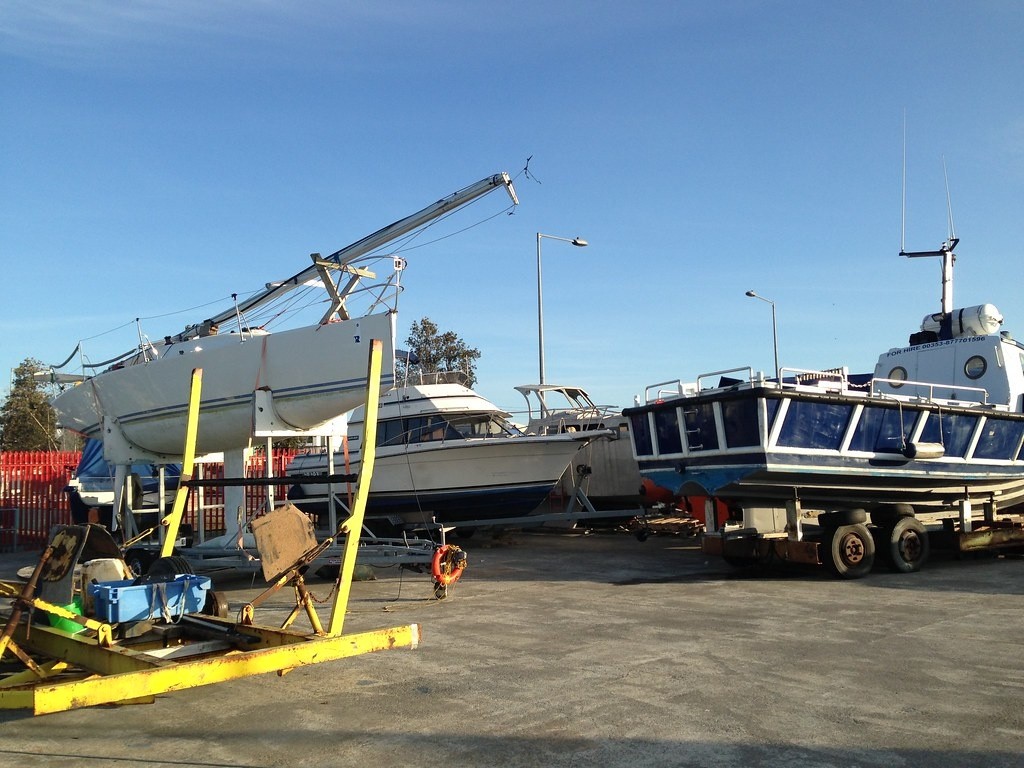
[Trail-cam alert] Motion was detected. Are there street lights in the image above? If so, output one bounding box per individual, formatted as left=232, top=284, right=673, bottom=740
left=536, top=232, right=590, bottom=420
left=745, top=290, right=780, bottom=379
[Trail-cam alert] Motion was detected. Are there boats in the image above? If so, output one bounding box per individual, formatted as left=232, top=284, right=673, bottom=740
left=286, top=370, right=617, bottom=525
left=44, top=168, right=521, bottom=464
left=622, top=234, right=1024, bottom=513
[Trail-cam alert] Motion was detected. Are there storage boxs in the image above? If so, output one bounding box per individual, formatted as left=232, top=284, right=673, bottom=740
left=86, top=574, right=212, bottom=621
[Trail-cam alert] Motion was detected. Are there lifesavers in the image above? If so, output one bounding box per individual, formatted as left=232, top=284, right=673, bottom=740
left=431, top=544, right=464, bottom=584
